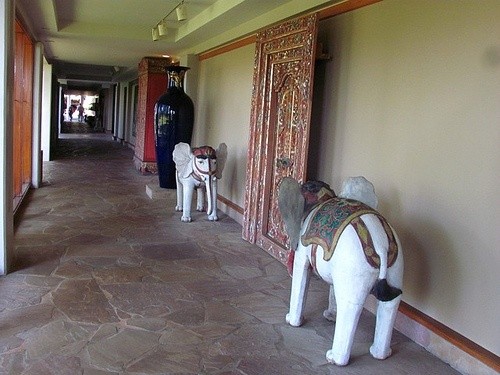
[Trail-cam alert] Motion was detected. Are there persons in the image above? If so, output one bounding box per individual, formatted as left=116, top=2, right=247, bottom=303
left=68, top=104, right=76, bottom=120
left=76, top=103, right=85, bottom=123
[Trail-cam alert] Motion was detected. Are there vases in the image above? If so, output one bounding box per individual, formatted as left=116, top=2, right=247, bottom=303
left=151, top=65, right=196, bottom=190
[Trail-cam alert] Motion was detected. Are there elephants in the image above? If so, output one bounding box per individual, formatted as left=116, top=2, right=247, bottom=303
left=277, top=176, right=404, bottom=366
left=172, top=142, right=228, bottom=223
